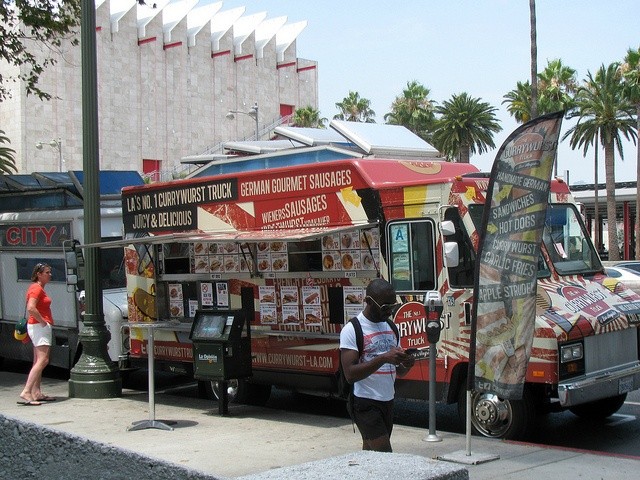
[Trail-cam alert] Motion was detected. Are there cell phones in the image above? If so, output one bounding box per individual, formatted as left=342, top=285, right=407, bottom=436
left=404, top=348, right=417, bottom=354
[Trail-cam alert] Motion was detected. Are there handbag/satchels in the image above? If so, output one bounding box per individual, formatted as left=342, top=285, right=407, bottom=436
left=21, top=334, right=31, bottom=344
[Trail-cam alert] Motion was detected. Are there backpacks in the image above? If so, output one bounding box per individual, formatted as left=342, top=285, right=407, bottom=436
left=332, top=316, right=399, bottom=414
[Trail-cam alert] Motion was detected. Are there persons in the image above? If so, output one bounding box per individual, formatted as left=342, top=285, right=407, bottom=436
left=339, top=279, right=415, bottom=452
left=19, top=262, right=56, bottom=405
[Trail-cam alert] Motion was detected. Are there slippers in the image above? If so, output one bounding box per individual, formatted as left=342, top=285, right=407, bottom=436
left=35, top=396, right=56, bottom=401
left=17, top=400, right=41, bottom=406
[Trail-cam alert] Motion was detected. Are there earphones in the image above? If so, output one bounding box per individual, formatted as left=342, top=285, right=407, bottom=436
left=369, top=301, right=371, bottom=305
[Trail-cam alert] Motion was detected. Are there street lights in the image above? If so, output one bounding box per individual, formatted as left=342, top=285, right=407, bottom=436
left=225, top=101, right=259, bottom=141
left=35, top=136, right=62, bottom=172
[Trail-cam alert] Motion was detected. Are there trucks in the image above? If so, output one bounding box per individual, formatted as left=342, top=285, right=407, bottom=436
left=0, top=170, right=150, bottom=382
left=122, top=121, right=640, bottom=436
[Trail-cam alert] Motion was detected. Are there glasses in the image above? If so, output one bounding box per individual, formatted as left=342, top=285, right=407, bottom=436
left=37, top=262, right=46, bottom=271
left=366, top=295, right=401, bottom=312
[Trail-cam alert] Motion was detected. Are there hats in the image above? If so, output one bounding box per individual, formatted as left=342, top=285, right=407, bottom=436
left=14, top=319, right=27, bottom=341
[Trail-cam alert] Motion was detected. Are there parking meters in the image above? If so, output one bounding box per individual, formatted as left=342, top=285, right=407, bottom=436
left=423, top=290, right=443, bottom=443
